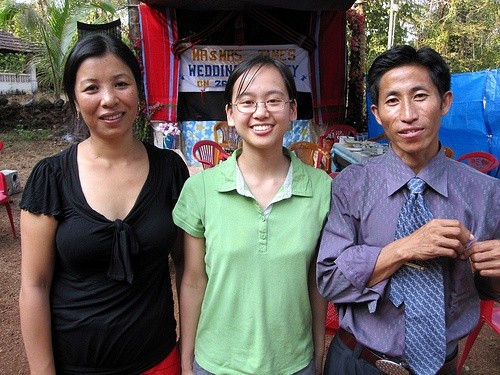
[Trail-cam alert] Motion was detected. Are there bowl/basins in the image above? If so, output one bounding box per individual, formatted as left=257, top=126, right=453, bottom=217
left=346, top=141, right=360, bottom=147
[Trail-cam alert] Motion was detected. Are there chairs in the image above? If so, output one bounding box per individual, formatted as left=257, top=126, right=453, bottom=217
left=455, top=300, right=500, bottom=375
left=0, top=140, right=17, bottom=240
left=214, top=121, right=243, bottom=165
left=456, top=152, right=499, bottom=173
left=193, top=140, right=230, bottom=171
left=288, top=140, right=332, bottom=174
left=328, top=172, right=341, bottom=180
left=317, top=124, right=358, bottom=171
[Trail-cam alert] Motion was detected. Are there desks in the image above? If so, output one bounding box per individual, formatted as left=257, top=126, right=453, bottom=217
left=329, top=141, right=389, bottom=174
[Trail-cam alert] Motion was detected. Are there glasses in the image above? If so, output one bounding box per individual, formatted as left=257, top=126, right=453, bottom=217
left=228, top=97, right=293, bottom=114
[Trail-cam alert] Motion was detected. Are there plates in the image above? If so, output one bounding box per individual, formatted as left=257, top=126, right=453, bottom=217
left=346, top=141, right=390, bottom=160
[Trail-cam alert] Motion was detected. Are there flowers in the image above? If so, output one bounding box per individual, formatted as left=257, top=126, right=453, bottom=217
left=126, top=32, right=167, bottom=142
left=155, top=122, right=181, bottom=138
left=346, top=7, right=366, bottom=133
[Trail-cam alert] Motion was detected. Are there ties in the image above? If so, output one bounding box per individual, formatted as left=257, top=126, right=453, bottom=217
left=389, top=180, right=446, bottom=375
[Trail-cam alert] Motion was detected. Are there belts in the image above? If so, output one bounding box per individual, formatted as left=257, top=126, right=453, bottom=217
left=338, top=327, right=458, bottom=375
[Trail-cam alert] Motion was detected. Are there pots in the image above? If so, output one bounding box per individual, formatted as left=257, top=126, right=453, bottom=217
left=337, top=136, right=354, bottom=143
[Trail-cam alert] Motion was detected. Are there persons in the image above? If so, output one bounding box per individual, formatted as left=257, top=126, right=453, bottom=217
left=172, top=55, right=333, bottom=375
left=19, top=33, right=190, bottom=375
left=317, top=45, right=500, bottom=375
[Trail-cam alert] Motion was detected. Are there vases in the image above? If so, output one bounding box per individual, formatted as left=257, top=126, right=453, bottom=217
left=163, top=134, right=176, bottom=149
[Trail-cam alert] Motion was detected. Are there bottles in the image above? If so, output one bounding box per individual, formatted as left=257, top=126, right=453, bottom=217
left=356, top=133, right=360, bottom=141
left=362, top=132, right=365, bottom=142
